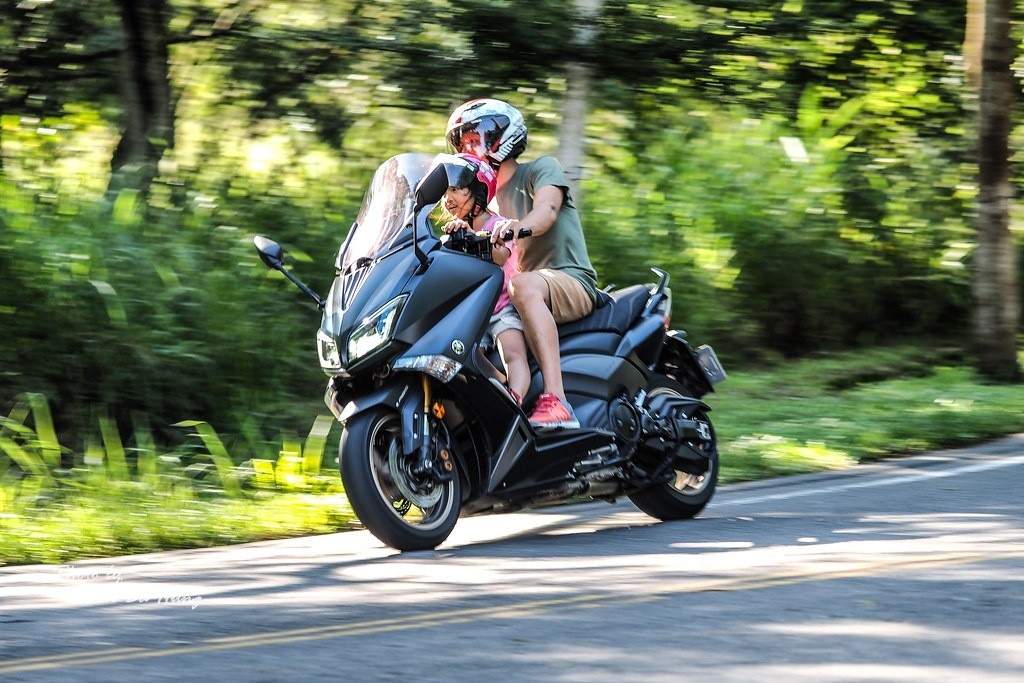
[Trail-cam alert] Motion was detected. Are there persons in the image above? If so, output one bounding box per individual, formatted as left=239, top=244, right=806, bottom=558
left=439, top=98, right=598, bottom=429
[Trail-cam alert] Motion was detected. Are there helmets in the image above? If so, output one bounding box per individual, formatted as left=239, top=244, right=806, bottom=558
left=445, top=99, right=527, bottom=165
left=444, top=153, right=497, bottom=207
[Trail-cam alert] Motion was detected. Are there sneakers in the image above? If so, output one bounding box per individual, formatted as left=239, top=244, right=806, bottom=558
left=527, top=393, right=580, bottom=429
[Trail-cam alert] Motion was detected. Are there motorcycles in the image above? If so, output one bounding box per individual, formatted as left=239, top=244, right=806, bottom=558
left=252, top=152, right=730, bottom=553
